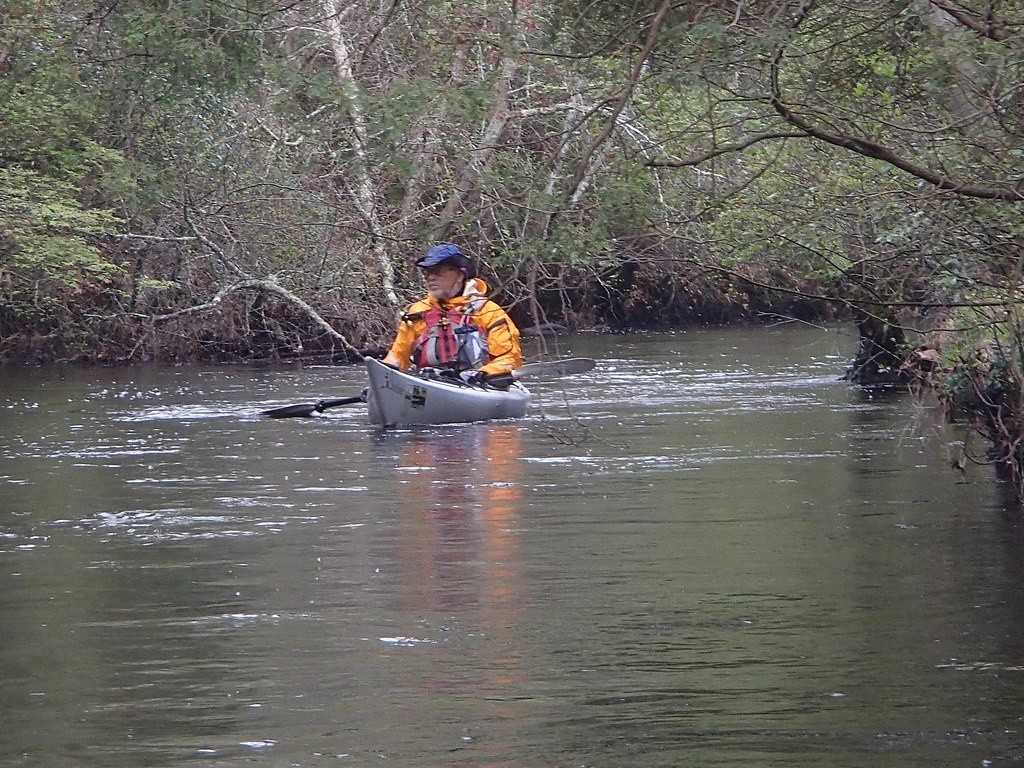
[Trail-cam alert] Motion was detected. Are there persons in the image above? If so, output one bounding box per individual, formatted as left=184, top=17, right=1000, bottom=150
left=360, top=246, right=522, bottom=403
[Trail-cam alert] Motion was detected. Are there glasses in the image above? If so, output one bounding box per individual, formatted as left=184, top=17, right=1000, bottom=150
left=423, top=266, right=459, bottom=276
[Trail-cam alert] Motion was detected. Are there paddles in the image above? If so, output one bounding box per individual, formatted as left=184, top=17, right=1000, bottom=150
left=260, top=357, right=596, bottom=419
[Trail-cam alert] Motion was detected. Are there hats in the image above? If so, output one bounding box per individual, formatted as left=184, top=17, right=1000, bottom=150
left=414, top=242, right=471, bottom=268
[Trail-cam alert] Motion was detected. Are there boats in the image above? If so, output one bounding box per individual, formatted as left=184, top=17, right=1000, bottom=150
left=362, top=355, right=532, bottom=426
